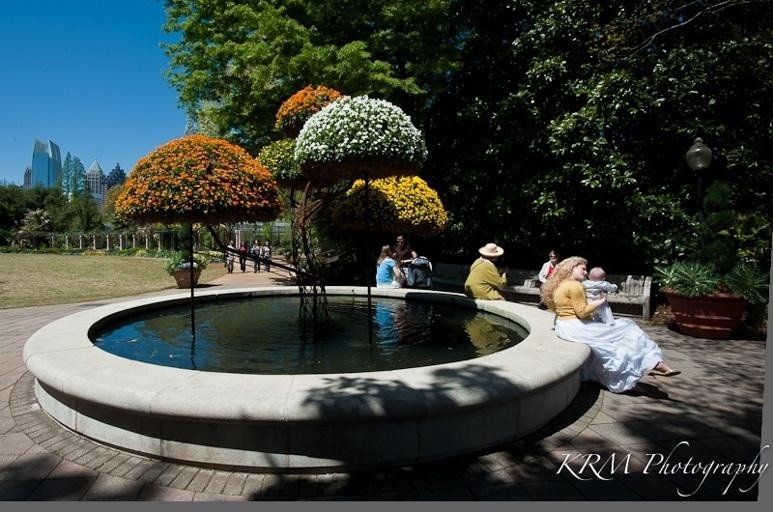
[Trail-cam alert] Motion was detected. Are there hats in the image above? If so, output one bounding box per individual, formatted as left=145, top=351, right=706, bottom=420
left=478, top=243, right=504, bottom=257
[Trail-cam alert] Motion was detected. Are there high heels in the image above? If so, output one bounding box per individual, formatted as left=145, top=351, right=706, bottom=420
left=648, top=369, right=681, bottom=379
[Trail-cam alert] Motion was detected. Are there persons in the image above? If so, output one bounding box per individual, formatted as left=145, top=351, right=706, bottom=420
left=374, top=244, right=406, bottom=288
left=225, top=239, right=234, bottom=274
left=580, top=266, right=619, bottom=326
left=373, top=299, right=510, bottom=356
left=538, top=255, right=682, bottom=395
left=238, top=241, right=248, bottom=272
left=392, top=234, right=419, bottom=279
left=538, top=249, right=561, bottom=284
left=463, top=242, right=508, bottom=301
left=262, top=240, right=272, bottom=273
left=250, top=239, right=262, bottom=275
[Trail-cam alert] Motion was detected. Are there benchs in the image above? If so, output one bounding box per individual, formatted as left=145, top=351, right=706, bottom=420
left=424, top=258, right=656, bottom=320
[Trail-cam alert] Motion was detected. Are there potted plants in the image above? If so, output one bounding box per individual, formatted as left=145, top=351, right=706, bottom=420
left=161, top=222, right=207, bottom=289
left=650, top=249, right=769, bottom=341
